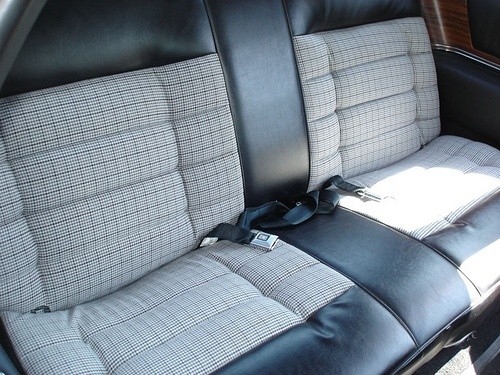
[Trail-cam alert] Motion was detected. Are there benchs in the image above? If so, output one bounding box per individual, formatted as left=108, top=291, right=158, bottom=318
left=0, top=0, right=500, bottom=375
